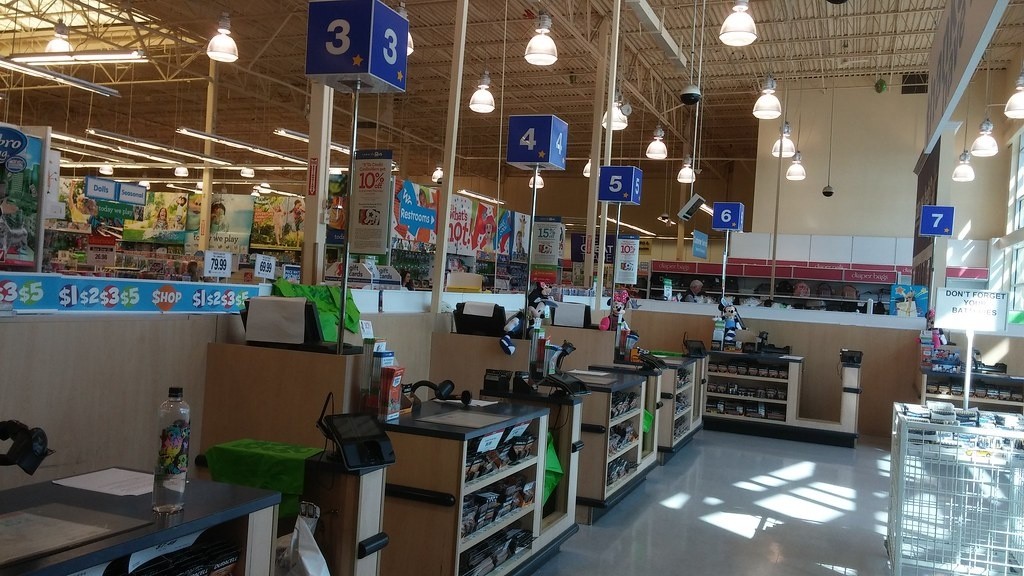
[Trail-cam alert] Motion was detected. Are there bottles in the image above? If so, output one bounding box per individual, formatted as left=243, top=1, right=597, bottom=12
left=153, top=387, right=192, bottom=513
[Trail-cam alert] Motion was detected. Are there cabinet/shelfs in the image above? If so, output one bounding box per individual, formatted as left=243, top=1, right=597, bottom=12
left=565, top=368, right=648, bottom=504
left=379, top=396, right=578, bottom=576
left=0, top=465, right=282, bottom=576
left=704, top=351, right=805, bottom=424
left=611, top=358, right=699, bottom=450
left=913, top=367, right=1024, bottom=416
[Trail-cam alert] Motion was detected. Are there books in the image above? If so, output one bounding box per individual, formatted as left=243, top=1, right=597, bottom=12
left=363, top=338, right=405, bottom=420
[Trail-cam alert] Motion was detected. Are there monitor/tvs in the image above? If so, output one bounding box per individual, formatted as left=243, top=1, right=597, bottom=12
left=641, top=354, right=668, bottom=371
left=244, top=298, right=326, bottom=346
left=453, top=304, right=508, bottom=338
left=323, top=414, right=396, bottom=471
left=547, top=372, right=592, bottom=396
left=840, top=350, right=862, bottom=364
left=734, top=308, right=748, bottom=331
left=685, top=339, right=707, bottom=357
left=550, top=303, right=592, bottom=328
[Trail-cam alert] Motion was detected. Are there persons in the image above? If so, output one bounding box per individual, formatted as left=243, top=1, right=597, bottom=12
left=69, top=180, right=92, bottom=228
left=267, top=200, right=287, bottom=246
left=680, top=280, right=704, bottom=303
left=399, top=271, right=414, bottom=291
left=153, top=208, right=168, bottom=230
left=288, top=199, right=305, bottom=247
left=513, top=231, right=525, bottom=262
left=181, top=262, right=198, bottom=282
left=174, top=197, right=187, bottom=230
left=417, top=189, right=435, bottom=244
left=211, top=202, right=227, bottom=233
left=478, top=215, right=497, bottom=252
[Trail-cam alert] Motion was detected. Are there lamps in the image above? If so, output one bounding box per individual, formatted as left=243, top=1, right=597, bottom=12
left=952, top=30, right=1023, bottom=183
left=430, top=0, right=696, bottom=192
left=44, top=123, right=398, bottom=198
left=719, top=0, right=807, bottom=181
left=45, top=0, right=75, bottom=52
left=397, top=0, right=414, bottom=56
left=206, top=0, right=239, bottom=63
left=0, top=55, right=123, bottom=98
left=8, top=49, right=150, bottom=66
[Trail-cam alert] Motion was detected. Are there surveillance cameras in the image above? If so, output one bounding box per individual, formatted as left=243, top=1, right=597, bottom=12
left=822, top=186, right=835, bottom=197
left=680, top=84, right=700, bottom=105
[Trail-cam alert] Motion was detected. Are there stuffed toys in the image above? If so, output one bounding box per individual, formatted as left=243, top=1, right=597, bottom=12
left=499, top=289, right=558, bottom=355
left=535, top=281, right=552, bottom=297
left=713, top=298, right=742, bottom=341
left=599, top=290, right=631, bottom=360
left=914, top=308, right=947, bottom=348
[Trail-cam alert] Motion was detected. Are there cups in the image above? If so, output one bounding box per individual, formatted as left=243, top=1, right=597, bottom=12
left=932, top=329, right=940, bottom=348
